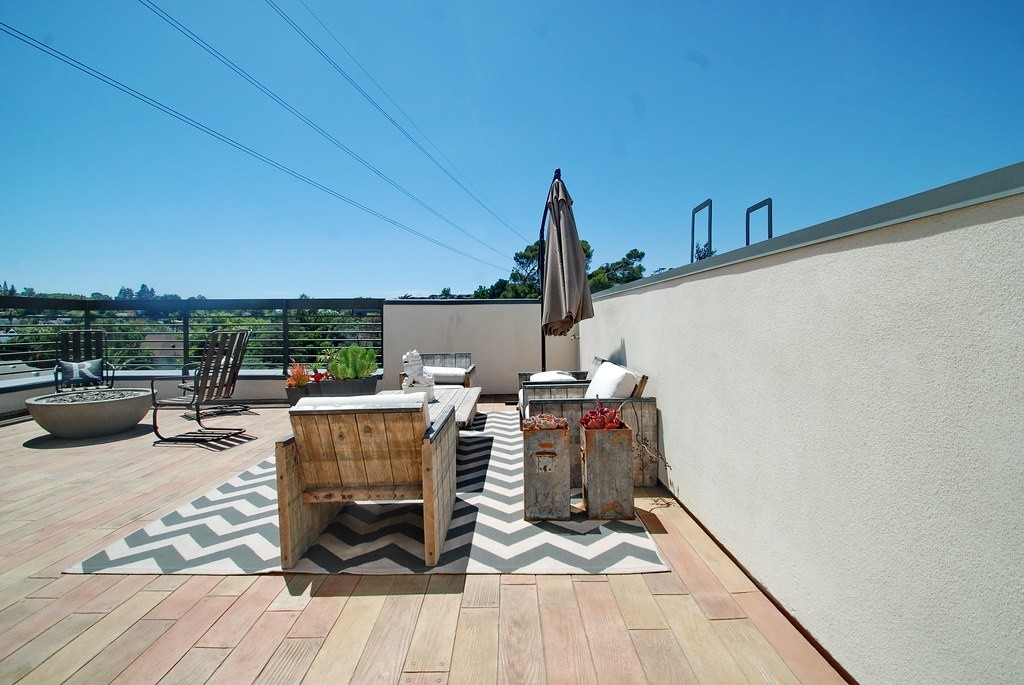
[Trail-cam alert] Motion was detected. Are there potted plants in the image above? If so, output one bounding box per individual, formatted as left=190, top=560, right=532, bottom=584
left=578, top=394, right=634, bottom=520
left=285, top=342, right=379, bottom=407
left=522, top=413, right=571, bottom=521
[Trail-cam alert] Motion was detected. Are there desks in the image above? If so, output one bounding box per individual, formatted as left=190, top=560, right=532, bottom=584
left=375, top=386, right=482, bottom=427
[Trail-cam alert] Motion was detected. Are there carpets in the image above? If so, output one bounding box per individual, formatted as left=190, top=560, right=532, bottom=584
left=61, top=408, right=671, bottom=574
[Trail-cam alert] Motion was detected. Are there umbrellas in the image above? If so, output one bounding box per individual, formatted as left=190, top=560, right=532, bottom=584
left=538, top=168, right=595, bottom=372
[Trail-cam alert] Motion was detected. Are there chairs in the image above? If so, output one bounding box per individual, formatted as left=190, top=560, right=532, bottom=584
left=150, top=331, right=249, bottom=444
left=518, top=357, right=658, bottom=487
left=399, top=352, right=476, bottom=390
left=275, top=392, right=457, bottom=569
left=181, top=328, right=253, bottom=415
left=54, top=329, right=114, bottom=392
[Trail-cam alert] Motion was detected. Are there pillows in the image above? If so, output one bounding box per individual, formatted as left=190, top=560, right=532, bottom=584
left=59, top=358, right=104, bottom=388
left=585, top=361, right=637, bottom=400
left=424, top=366, right=467, bottom=384
left=530, top=370, right=577, bottom=381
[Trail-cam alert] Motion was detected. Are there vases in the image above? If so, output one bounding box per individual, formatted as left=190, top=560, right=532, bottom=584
left=25, top=388, right=158, bottom=439
